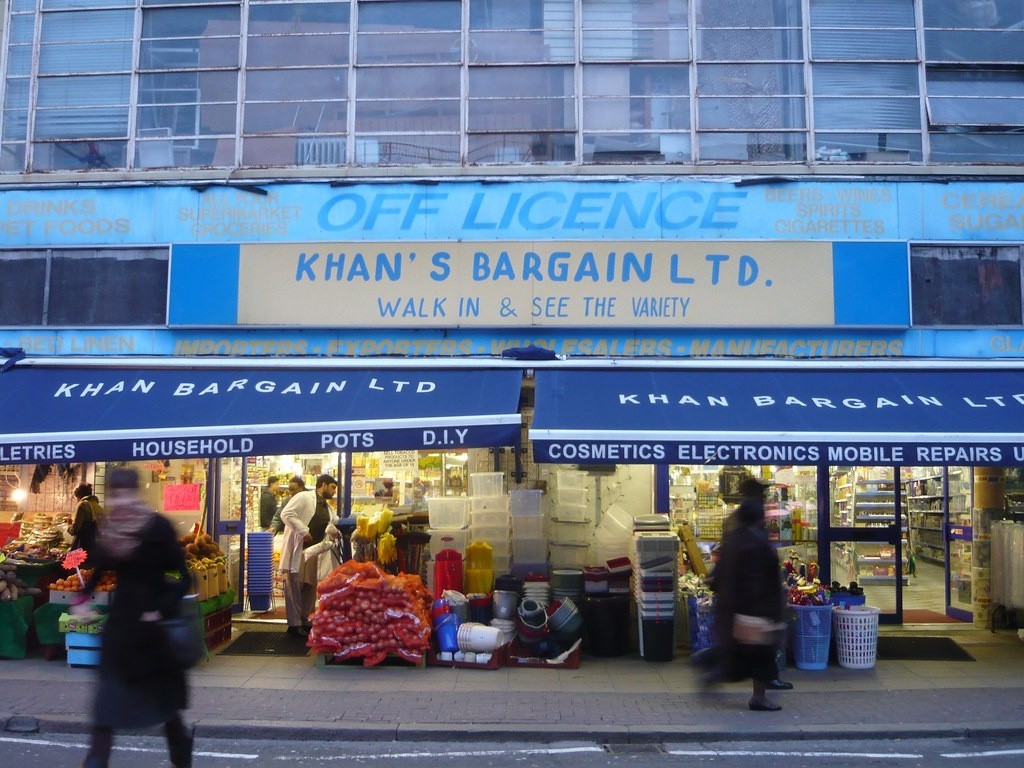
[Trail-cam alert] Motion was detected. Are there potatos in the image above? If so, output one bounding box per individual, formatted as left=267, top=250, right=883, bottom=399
left=0, top=565, right=41, bottom=600
left=312, top=562, right=431, bottom=651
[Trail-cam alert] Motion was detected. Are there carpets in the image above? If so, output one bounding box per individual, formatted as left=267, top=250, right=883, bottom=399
left=250, top=604, right=319, bottom=619
left=216, top=631, right=312, bottom=656
left=876, top=637, right=976, bottom=661
left=878, top=608, right=964, bottom=623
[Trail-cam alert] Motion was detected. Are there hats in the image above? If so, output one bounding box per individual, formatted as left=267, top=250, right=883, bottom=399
left=739, top=479, right=770, bottom=494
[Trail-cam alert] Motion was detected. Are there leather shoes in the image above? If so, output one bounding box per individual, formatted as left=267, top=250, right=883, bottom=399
left=766, top=678, right=793, bottom=689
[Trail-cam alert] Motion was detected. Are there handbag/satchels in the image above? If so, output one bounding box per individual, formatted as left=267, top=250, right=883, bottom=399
left=732, top=613, right=789, bottom=646
left=155, top=618, right=204, bottom=676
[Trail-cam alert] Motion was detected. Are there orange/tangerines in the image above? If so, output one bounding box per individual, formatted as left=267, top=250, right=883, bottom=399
left=48, top=569, right=116, bottom=592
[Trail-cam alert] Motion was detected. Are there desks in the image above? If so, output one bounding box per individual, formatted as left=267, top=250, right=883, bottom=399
left=0, top=595, right=33, bottom=659
left=33, top=603, right=109, bottom=659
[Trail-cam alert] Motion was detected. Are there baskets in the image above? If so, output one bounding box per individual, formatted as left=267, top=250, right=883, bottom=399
left=787, top=602, right=834, bottom=671
left=688, top=594, right=721, bottom=663
left=832, top=605, right=880, bottom=669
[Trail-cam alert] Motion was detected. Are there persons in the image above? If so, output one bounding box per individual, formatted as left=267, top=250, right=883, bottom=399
left=65, top=468, right=195, bottom=768
left=258, top=475, right=345, bottom=638
left=689, top=481, right=794, bottom=712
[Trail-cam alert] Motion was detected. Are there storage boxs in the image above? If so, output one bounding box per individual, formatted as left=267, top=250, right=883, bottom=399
left=425, top=498, right=472, bottom=593
left=471, top=472, right=513, bottom=587
left=592, top=504, right=633, bottom=567
left=509, top=489, right=549, bottom=578
left=49, top=590, right=115, bottom=605
left=669, top=475, right=694, bottom=500
left=550, top=470, right=593, bottom=569
left=5, top=557, right=55, bottom=588
left=634, top=532, right=681, bottom=572
left=248, top=532, right=274, bottom=610
left=584, top=557, right=633, bottom=597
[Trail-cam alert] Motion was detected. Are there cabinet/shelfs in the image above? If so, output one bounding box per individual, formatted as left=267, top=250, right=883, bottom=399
left=246, top=451, right=468, bottom=515
left=908, top=470, right=975, bottom=567
left=669, top=465, right=818, bottom=523
left=833, top=479, right=910, bottom=586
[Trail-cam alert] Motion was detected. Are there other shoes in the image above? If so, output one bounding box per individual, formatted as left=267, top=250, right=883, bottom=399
left=749, top=697, right=782, bottom=711
left=288, top=625, right=312, bottom=638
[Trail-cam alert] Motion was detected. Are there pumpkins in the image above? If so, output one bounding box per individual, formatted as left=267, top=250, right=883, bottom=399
left=179, top=534, right=222, bottom=558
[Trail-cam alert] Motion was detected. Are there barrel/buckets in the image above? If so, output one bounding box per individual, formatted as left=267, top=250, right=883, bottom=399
left=432, top=575, right=551, bottom=653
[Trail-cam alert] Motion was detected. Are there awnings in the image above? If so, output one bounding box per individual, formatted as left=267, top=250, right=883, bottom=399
left=530, top=368, right=1024, bottom=467
left=0, top=366, right=525, bottom=465
